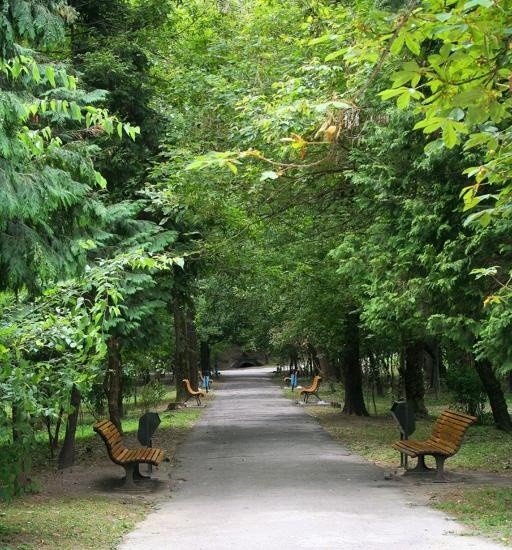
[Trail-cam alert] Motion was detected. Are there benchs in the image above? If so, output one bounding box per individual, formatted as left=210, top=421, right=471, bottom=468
left=274, top=366, right=282, bottom=375
left=183, top=379, right=205, bottom=407
left=284, top=377, right=291, bottom=387
left=198, top=370, right=213, bottom=388
left=391, top=409, right=478, bottom=482
left=299, top=375, right=323, bottom=405
left=93, top=419, right=163, bottom=491
